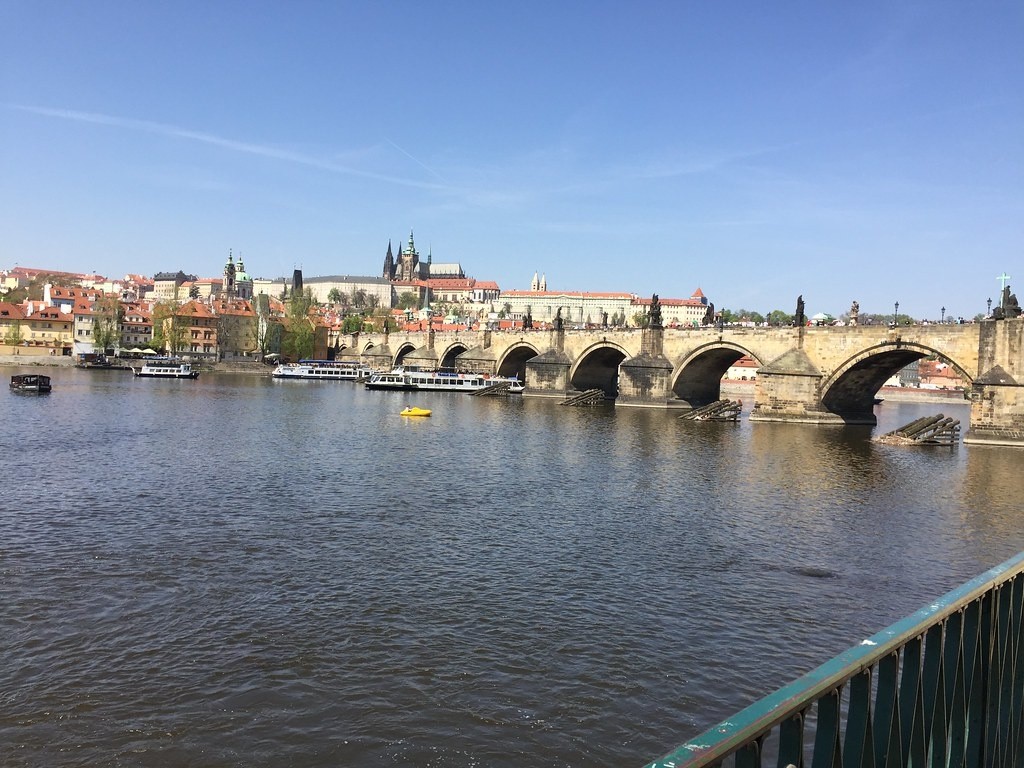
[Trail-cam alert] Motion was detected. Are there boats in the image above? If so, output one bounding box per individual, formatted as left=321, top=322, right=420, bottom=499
left=364, top=366, right=525, bottom=390
left=9, top=374, right=52, bottom=394
left=272, top=360, right=364, bottom=378
left=399, top=407, right=433, bottom=415
left=130, top=363, right=200, bottom=377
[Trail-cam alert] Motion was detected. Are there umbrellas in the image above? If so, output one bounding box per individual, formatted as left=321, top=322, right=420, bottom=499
left=119, top=347, right=157, bottom=356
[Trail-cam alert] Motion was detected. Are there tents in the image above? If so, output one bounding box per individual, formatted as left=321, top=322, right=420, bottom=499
left=812, top=313, right=836, bottom=320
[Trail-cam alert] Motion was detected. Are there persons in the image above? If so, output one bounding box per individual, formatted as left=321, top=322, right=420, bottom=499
left=50, top=348, right=56, bottom=356
left=816, top=320, right=819, bottom=326
left=912, top=319, right=951, bottom=325
left=406, top=405, right=411, bottom=412
left=806, top=319, right=812, bottom=326
left=954, top=317, right=964, bottom=324
left=822, top=319, right=825, bottom=326
left=667, top=320, right=698, bottom=328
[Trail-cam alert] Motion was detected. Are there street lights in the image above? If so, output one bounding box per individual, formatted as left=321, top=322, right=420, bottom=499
left=721, top=307, right=726, bottom=326
left=941, top=306, right=946, bottom=325
left=985, top=297, right=993, bottom=317
left=895, top=300, right=900, bottom=325
